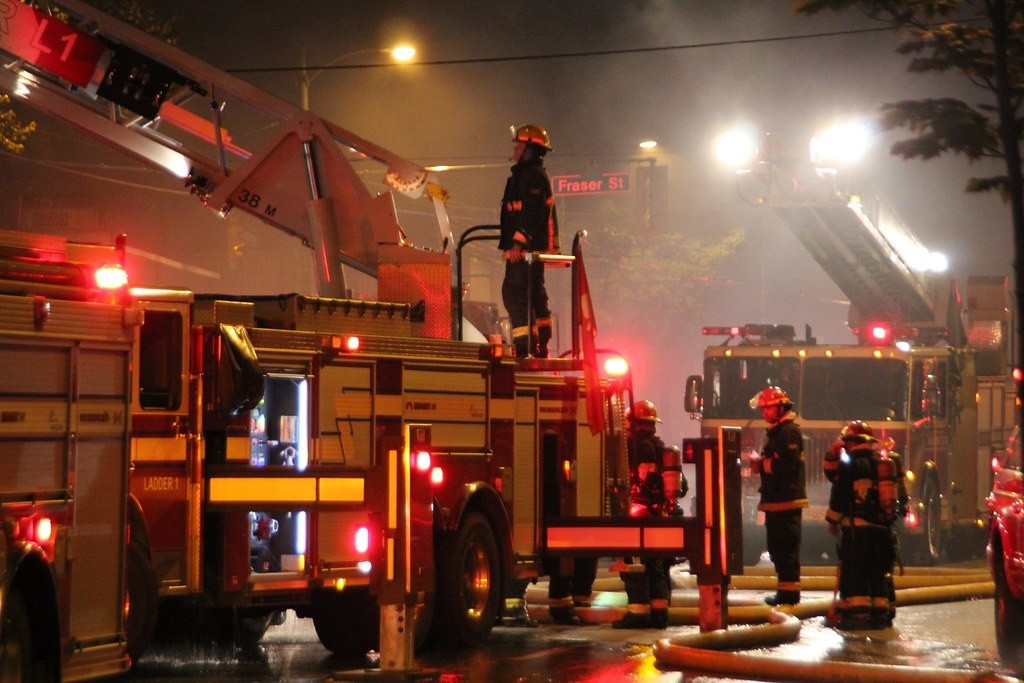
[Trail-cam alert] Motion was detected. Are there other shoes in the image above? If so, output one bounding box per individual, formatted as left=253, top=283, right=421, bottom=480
left=650, top=615, right=668, bottom=630
left=552, top=614, right=582, bottom=626
left=611, top=611, right=650, bottom=630
left=764, top=593, right=798, bottom=607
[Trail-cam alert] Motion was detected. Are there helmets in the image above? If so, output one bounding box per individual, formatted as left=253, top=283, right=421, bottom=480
left=757, top=385, right=796, bottom=408
left=627, top=399, right=664, bottom=424
left=837, top=418, right=879, bottom=444
left=511, top=123, right=555, bottom=154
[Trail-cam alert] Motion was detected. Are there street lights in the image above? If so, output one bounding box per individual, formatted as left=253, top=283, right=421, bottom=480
left=297, top=34, right=416, bottom=115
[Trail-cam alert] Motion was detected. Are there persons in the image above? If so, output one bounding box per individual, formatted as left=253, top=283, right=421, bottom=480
left=497, top=125, right=560, bottom=359
left=495, top=386, right=908, bottom=630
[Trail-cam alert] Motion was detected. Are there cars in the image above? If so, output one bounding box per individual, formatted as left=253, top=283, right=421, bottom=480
left=989, top=420, right=1024, bottom=667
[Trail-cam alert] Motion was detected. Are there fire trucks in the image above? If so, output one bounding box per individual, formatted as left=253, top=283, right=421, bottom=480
left=0, top=0, right=688, bottom=683
left=683, top=154, right=1017, bottom=566
